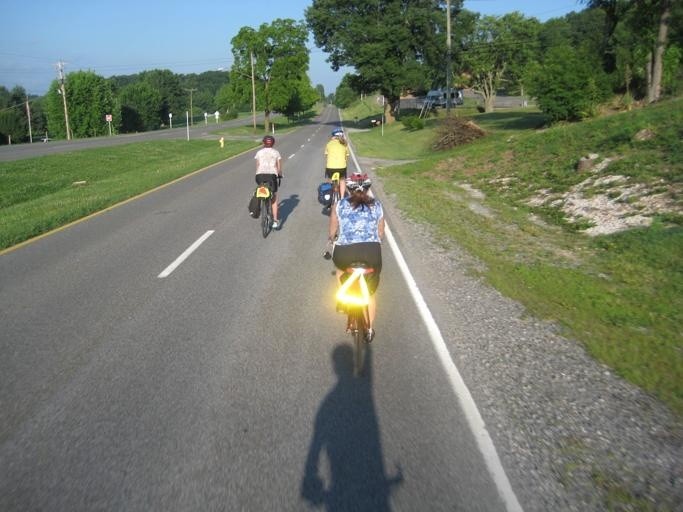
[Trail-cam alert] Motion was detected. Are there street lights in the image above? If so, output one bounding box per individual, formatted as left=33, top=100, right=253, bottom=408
left=218, top=66, right=257, bottom=129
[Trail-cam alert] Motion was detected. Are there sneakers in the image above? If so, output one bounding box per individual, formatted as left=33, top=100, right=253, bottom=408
left=272, top=219, right=281, bottom=228
left=363, top=329, right=375, bottom=343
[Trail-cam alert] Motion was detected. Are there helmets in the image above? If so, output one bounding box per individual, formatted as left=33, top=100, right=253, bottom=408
left=331, top=128, right=343, bottom=135
left=346, top=173, right=372, bottom=189
left=263, top=136, right=275, bottom=146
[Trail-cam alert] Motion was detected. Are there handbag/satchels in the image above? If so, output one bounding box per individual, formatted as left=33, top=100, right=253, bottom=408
left=249, top=191, right=261, bottom=218
left=318, top=183, right=332, bottom=206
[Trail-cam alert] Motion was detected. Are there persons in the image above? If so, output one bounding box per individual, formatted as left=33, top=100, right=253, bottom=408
left=254, top=135, right=281, bottom=228
left=324, top=128, right=350, bottom=200
left=329, top=172, right=384, bottom=344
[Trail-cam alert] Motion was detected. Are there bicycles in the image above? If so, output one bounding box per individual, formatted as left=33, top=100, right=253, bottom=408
left=254, top=173, right=284, bottom=239
left=331, top=173, right=341, bottom=204
left=322, top=233, right=377, bottom=376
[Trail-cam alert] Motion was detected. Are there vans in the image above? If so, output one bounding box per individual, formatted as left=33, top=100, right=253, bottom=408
left=420, top=86, right=465, bottom=109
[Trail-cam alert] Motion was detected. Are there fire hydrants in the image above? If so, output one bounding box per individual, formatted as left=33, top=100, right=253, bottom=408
left=219, top=136, right=225, bottom=148
